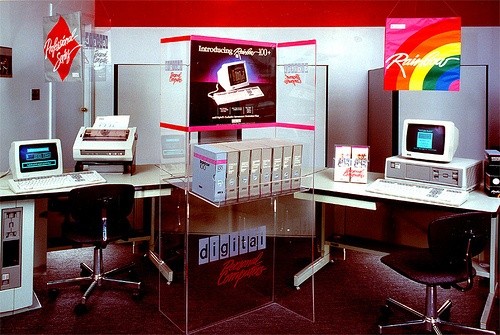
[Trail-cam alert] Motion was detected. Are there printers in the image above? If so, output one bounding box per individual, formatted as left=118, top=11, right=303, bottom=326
left=73, top=126, right=139, bottom=175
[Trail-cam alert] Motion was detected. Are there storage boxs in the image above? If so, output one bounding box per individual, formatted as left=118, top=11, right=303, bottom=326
left=192, top=139, right=304, bottom=204
left=483, top=149, right=500, bottom=198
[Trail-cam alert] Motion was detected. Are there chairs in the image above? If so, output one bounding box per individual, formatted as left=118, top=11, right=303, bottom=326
left=373, top=211, right=495, bottom=335
left=45, top=184, right=142, bottom=314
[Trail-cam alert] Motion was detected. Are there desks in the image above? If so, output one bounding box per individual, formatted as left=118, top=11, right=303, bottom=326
left=299, top=167, right=500, bottom=330
left=0, top=163, right=184, bottom=317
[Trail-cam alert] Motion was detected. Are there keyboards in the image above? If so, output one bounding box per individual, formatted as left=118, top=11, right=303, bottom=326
left=213, top=87, right=265, bottom=105
left=365, top=178, right=469, bottom=206
left=8, top=171, right=107, bottom=193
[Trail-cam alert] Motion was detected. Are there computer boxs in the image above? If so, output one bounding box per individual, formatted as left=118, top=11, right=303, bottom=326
left=0, top=198, right=35, bottom=313
left=384, top=155, right=483, bottom=193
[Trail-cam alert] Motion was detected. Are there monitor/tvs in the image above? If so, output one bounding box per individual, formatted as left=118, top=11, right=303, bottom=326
left=9, top=139, right=63, bottom=181
left=402, top=119, right=459, bottom=162
left=217, top=61, right=249, bottom=92
